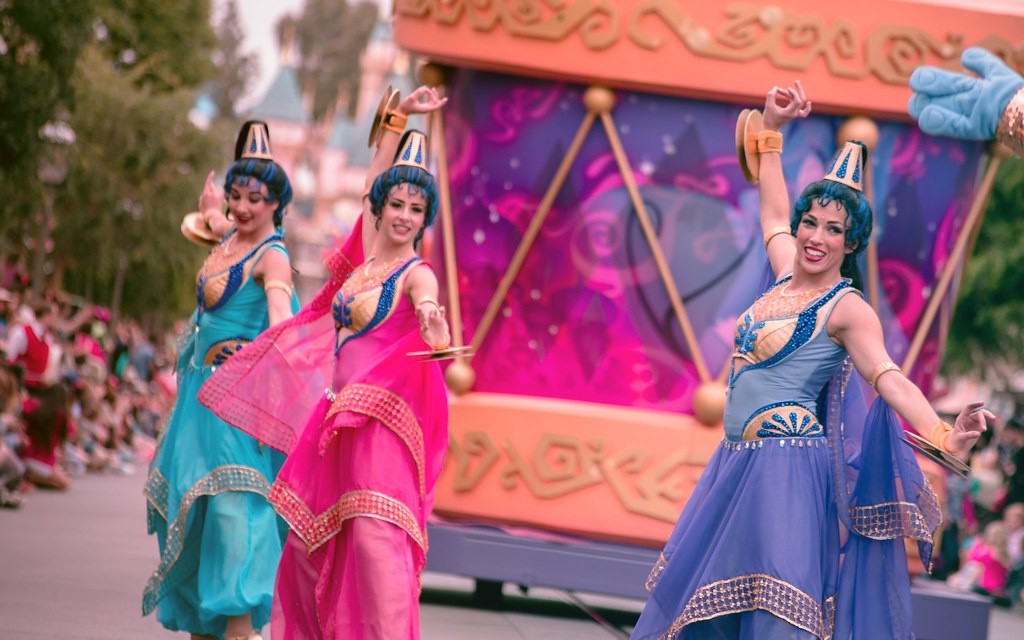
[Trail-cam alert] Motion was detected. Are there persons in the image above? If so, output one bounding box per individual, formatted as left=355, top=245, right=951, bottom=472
left=141, top=117, right=323, bottom=640
left=926, top=414, right=1024, bottom=607
left=0, top=254, right=179, bottom=508
left=197, top=87, right=451, bottom=640
left=631, top=78, right=997, bottom=640
